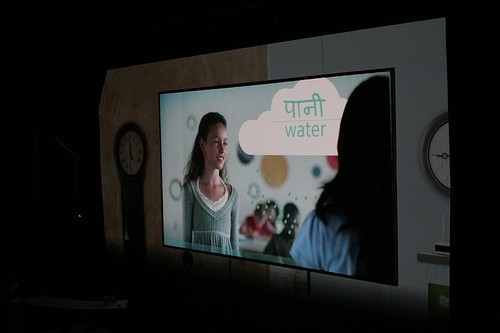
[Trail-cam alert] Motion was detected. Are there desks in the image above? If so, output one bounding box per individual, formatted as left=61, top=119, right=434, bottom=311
left=16, top=294, right=129, bottom=332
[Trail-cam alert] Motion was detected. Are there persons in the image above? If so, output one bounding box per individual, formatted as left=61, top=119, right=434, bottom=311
left=181, top=111, right=240, bottom=252
left=239, top=203, right=279, bottom=240
left=289, top=73, right=392, bottom=276
left=263, top=202, right=301, bottom=259
left=265, top=200, right=282, bottom=232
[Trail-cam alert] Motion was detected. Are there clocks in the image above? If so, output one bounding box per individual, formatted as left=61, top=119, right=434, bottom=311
left=113, top=121, right=147, bottom=182
left=421, top=113, right=451, bottom=194
